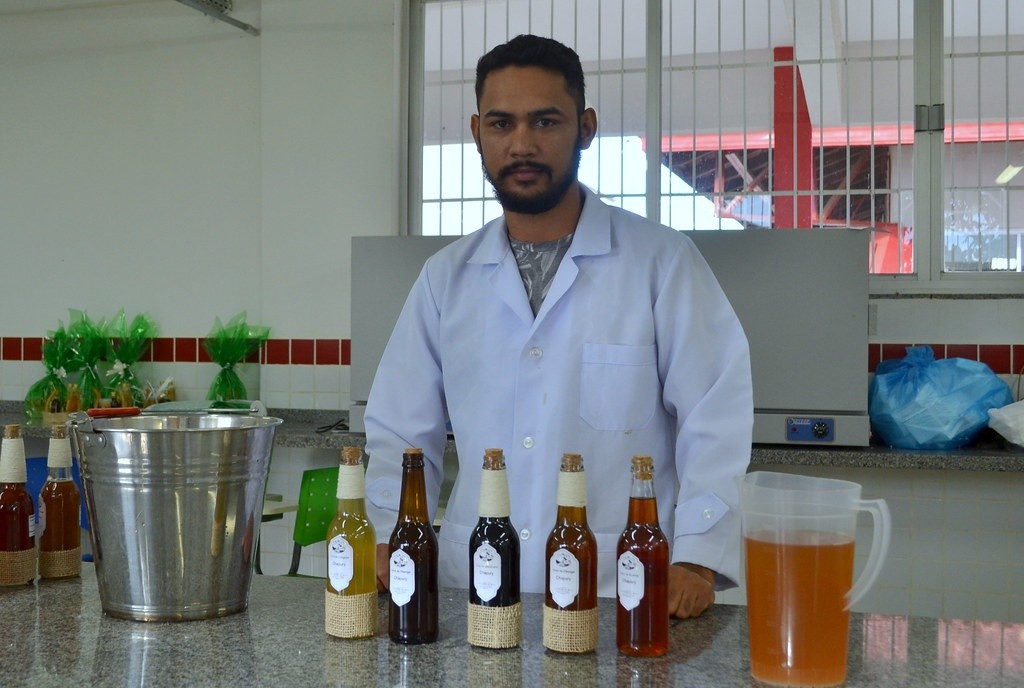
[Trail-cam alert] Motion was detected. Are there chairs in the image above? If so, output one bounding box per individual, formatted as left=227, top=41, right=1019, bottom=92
left=287, top=464, right=341, bottom=579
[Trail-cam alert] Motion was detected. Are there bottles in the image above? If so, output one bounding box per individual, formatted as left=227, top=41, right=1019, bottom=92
left=389, top=449, right=440, bottom=645
left=38, top=424, right=82, bottom=579
left=616, top=456, right=670, bottom=658
left=0, top=424, right=38, bottom=589
left=325, top=448, right=377, bottom=640
left=469, top=451, right=523, bottom=650
left=544, top=454, right=599, bottom=655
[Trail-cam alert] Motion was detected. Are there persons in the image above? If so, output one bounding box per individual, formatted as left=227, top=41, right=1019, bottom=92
left=364, top=35, right=755, bottom=620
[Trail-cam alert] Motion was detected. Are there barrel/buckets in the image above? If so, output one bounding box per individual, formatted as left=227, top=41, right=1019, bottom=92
left=72, top=407, right=284, bottom=622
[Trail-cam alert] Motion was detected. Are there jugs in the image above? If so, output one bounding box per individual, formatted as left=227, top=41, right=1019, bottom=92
left=738, top=472, right=892, bottom=688
